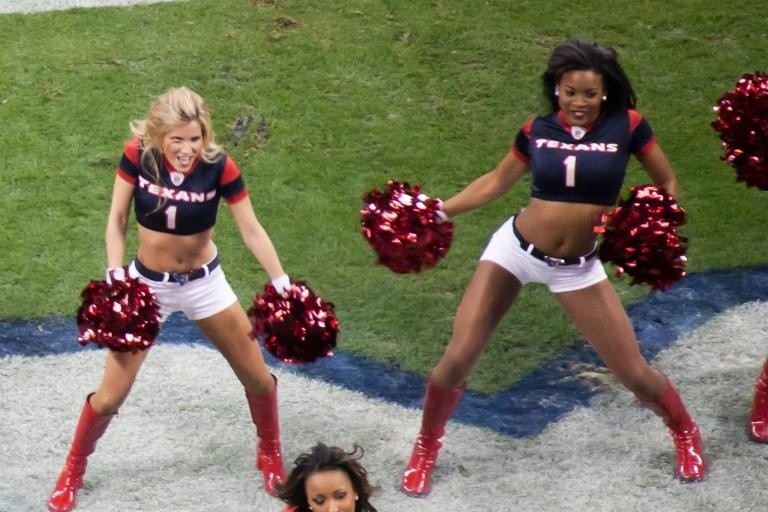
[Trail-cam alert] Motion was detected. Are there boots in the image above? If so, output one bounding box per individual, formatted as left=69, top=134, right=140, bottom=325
left=633, top=372, right=705, bottom=481
left=401, top=375, right=464, bottom=496
left=48, top=395, right=114, bottom=510
left=246, top=375, right=290, bottom=498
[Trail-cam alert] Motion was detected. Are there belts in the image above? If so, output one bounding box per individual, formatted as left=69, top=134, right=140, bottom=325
left=135, top=255, right=221, bottom=283
left=513, top=216, right=599, bottom=267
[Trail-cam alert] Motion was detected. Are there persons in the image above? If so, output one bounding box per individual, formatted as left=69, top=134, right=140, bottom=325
left=53, top=86, right=298, bottom=490
left=270, top=444, right=381, bottom=512
left=398, top=42, right=708, bottom=496
